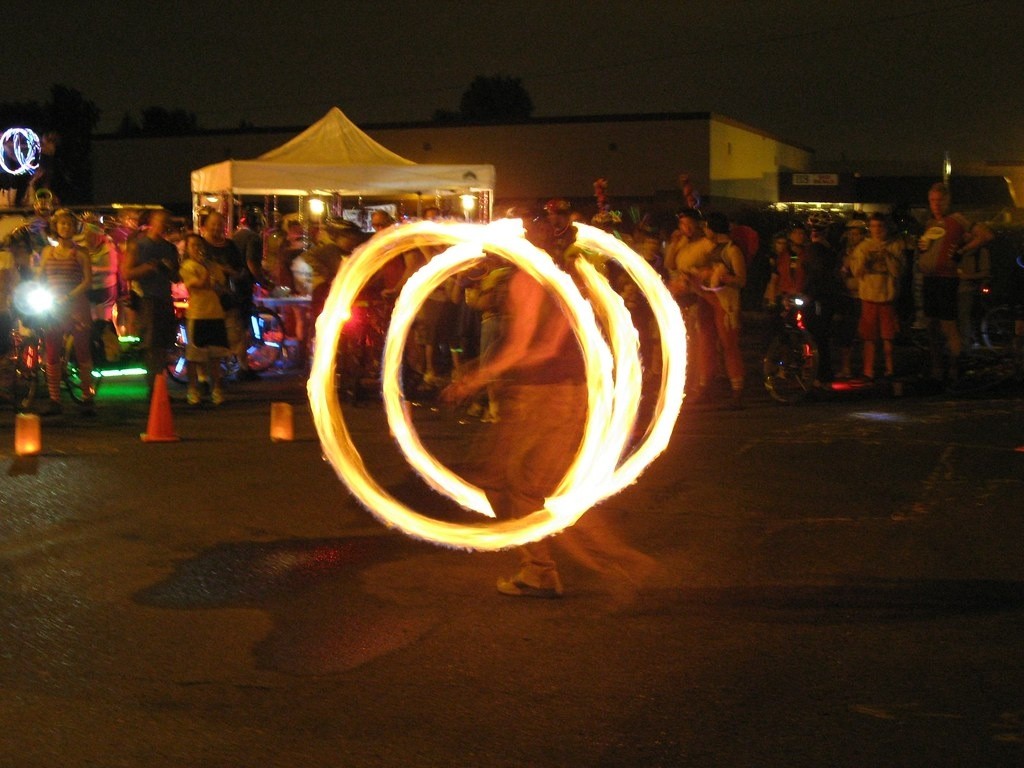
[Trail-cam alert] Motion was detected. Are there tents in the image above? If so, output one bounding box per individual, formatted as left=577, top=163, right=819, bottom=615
left=190, top=107, right=496, bottom=237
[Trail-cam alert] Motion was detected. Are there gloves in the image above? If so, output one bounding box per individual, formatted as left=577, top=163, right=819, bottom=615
left=951, top=249, right=965, bottom=263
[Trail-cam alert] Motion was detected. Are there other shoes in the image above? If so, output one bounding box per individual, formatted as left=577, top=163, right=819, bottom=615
left=79, top=398, right=99, bottom=418
left=689, top=384, right=714, bottom=403
left=718, top=395, right=747, bottom=412
left=851, top=372, right=876, bottom=386
left=494, top=565, right=564, bottom=598
left=185, top=386, right=200, bottom=406
left=479, top=412, right=503, bottom=424
left=209, top=388, right=224, bottom=404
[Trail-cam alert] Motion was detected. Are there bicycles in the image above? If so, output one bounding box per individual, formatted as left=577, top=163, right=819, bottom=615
left=759, top=290, right=819, bottom=406
left=8, top=262, right=109, bottom=412
left=163, top=279, right=287, bottom=386
left=977, top=283, right=1015, bottom=353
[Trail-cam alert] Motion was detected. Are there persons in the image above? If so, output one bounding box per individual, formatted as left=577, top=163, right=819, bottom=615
left=20, top=183, right=1024, bottom=599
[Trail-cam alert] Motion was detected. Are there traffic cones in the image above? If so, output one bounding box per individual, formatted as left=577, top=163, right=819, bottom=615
left=138, top=367, right=184, bottom=442
